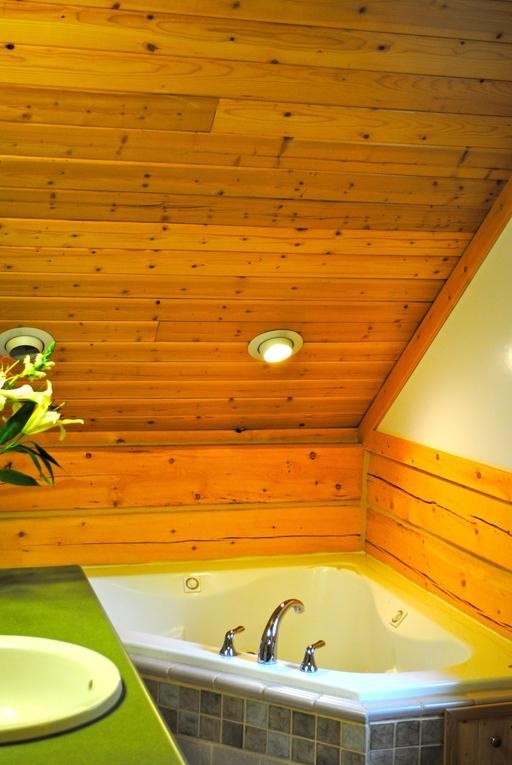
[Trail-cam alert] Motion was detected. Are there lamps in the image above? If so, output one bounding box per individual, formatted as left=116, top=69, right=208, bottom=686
left=247, top=329, right=304, bottom=364
left=1, top=325, right=56, bottom=365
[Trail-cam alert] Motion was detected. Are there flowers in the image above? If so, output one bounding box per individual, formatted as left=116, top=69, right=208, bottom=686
left=1, top=351, right=85, bottom=492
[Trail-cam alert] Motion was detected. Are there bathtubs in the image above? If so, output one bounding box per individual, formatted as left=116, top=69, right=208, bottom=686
left=80, top=550, right=512, bottom=704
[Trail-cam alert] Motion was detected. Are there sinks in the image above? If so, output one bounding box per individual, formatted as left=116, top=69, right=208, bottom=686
left=0, top=633, right=124, bottom=747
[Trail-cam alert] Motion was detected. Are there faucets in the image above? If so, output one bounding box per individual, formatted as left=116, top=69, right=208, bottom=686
left=255, top=598, right=306, bottom=665
